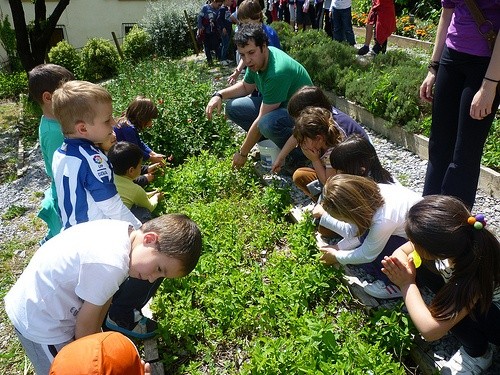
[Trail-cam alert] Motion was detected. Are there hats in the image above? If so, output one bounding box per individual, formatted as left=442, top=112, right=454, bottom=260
left=49, top=331, right=145, bottom=375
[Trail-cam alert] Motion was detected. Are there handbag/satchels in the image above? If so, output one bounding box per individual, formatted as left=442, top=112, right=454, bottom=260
left=196, top=25, right=206, bottom=42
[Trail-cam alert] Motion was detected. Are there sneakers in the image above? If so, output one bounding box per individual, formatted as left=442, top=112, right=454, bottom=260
left=438, top=343, right=494, bottom=375
left=106, top=308, right=162, bottom=339
left=364, top=279, right=403, bottom=298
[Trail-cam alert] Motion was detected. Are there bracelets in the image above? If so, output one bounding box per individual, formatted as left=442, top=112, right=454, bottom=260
left=484, top=77, right=500, bottom=82
left=238, top=148, right=248, bottom=157
left=212, top=92, right=222, bottom=98
left=428, top=60, right=440, bottom=71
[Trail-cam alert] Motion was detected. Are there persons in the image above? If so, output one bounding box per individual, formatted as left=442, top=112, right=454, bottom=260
left=310, top=132, right=401, bottom=239
left=380, top=194, right=500, bottom=375
left=195, top=0, right=356, bottom=97
left=2, top=64, right=203, bottom=375
left=357, top=0, right=396, bottom=56
left=269, top=85, right=371, bottom=173
left=205, top=23, right=312, bottom=171
left=310, top=174, right=425, bottom=299
left=422, top=0, right=500, bottom=212
left=291, top=105, right=348, bottom=203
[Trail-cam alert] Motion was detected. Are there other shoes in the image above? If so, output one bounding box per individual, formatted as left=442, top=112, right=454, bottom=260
left=280, top=157, right=314, bottom=175
left=221, top=58, right=234, bottom=65
left=357, top=46, right=369, bottom=55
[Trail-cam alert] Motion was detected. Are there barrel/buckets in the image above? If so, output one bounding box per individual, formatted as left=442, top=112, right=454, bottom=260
left=258, top=140, right=285, bottom=169
